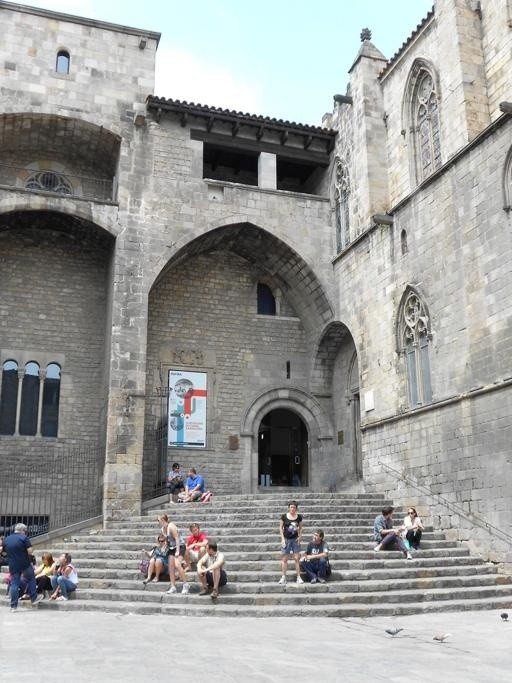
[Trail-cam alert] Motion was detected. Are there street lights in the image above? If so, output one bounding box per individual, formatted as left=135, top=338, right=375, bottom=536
left=141, top=560, right=149, bottom=573
left=161, top=544, right=170, bottom=556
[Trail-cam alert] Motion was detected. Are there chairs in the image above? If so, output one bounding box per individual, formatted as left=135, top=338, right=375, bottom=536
left=144, top=577, right=150, bottom=584
left=199, top=588, right=208, bottom=595
left=20, top=594, right=30, bottom=599
left=32, top=595, right=44, bottom=605
left=279, top=575, right=326, bottom=584
left=49, top=596, right=68, bottom=601
left=182, top=584, right=190, bottom=594
left=167, top=586, right=177, bottom=593
left=153, top=578, right=158, bottom=583
left=407, top=554, right=412, bottom=560
left=10, top=607, right=22, bottom=612
left=374, top=547, right=379, bottom=551
left=210, top=590, right=219, bottom=597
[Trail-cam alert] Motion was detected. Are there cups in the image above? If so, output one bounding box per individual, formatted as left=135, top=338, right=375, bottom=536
left=408, top=512, right=413, bottom=514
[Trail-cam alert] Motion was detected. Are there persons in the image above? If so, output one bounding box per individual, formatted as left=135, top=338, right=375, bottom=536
left=0, top=536, right=36, bottom=599
left=142, top=533, right=170, bottom=583
left=157, top=513, right=191, bottom=594
left=20, top=551, right=57, bottom=599
left=50, top=553, right=80, bottom=600
left=0, top=522, right=44, bottom=612
left=403, top=507, right=424, bottom=548
left=183, top=523, right=209, bottom=572
left=373, top=507, right=412, bottom=560
left=278, top=501, right=304, bottom=585
left=299, top=528, right=331, bottom=584
left=177, top=467, right=204, bottom=502
left=197, top=542, right=228, bottom=597
left=166, top=463, right=185, bottom=504
